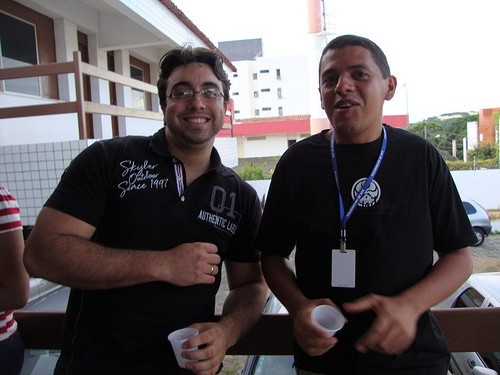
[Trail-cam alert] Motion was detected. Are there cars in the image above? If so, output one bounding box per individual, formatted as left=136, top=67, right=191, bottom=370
left=461, top=198, right=492, bottom=248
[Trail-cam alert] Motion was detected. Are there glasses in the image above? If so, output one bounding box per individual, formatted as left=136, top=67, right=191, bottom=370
left=166, top=88, right=227, bottom=102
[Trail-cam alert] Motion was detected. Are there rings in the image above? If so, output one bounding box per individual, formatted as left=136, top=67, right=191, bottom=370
left=210, top=264, right=215, bottom=275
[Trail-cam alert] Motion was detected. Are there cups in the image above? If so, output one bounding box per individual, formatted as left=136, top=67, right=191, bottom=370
left=312, top=305, right=344, bottom=339
left=167, top=328, right=200, bottom=367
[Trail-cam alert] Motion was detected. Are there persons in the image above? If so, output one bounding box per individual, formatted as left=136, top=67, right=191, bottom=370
left=21, top=47, right=271, bottom=375
left=0, top=185, right=30, bottom=374
left=254, top=35, right=479, bottom=375
